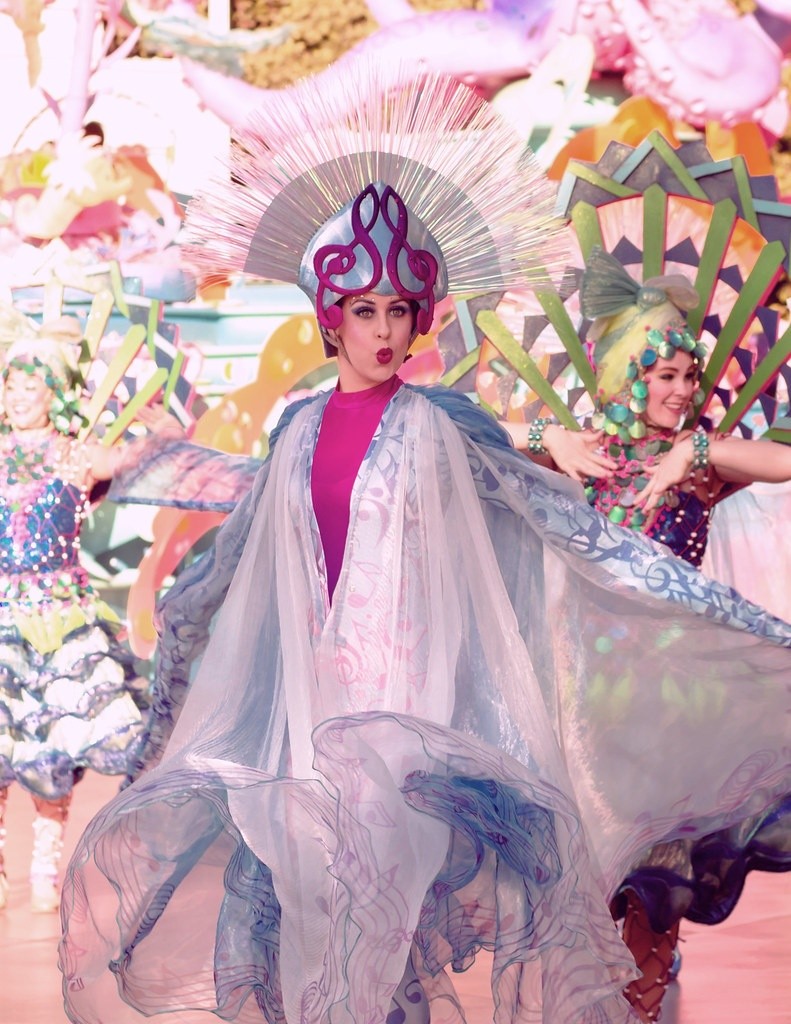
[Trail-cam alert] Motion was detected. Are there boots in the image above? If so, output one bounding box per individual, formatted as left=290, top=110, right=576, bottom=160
left=0, top=828, right=10, bottom=908
left=31, top=815, right=66, bottom=914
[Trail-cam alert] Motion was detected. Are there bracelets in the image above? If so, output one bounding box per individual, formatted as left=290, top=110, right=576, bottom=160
left=528, top=418, right=549, bottom=454
left=693, top=434, right=708, bottom=468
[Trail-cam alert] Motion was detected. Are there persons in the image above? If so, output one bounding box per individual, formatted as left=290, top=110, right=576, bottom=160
left=61, top=193, right=790, bottom=1024
left=494, top=305, right=790, bottom=1024
left=2, top=338, right=184, bottom=911
